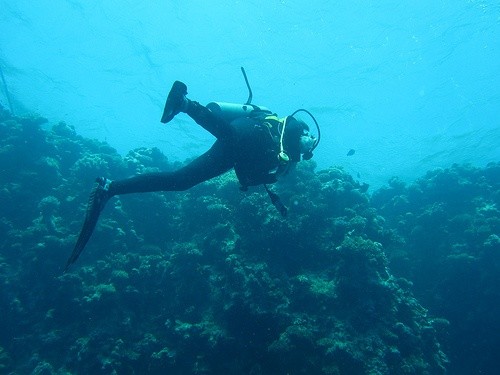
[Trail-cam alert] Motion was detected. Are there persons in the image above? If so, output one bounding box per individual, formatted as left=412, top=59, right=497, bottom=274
left=84, top=81, right=316, bottom=224
